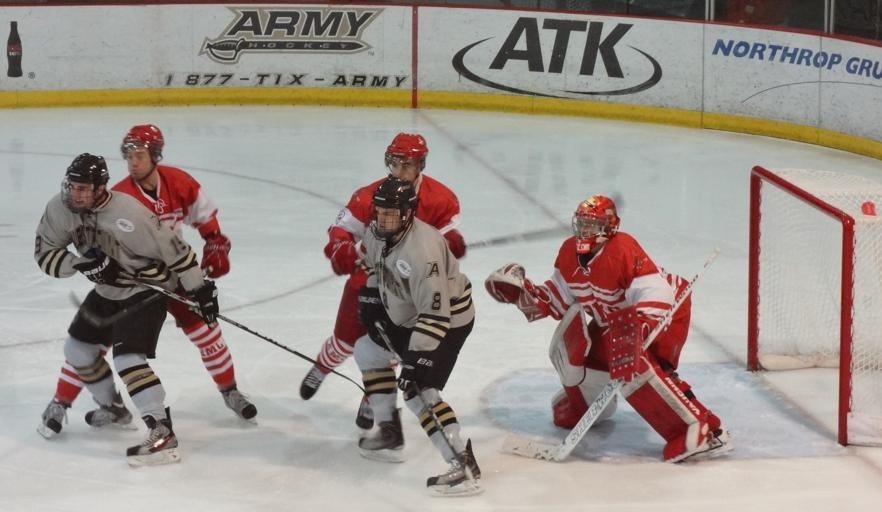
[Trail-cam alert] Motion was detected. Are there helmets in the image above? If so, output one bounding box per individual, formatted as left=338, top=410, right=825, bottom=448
left=384, top=132, right=429, bottom=171
left=121, top=124, right=164, bottom=163
left=370, top=178, right=428, bottom=239
left=61, top=153, right=108, bottom=210
left=571, top=195, right=621, bottom=247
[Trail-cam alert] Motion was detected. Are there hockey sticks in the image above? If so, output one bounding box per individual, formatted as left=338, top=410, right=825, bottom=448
left=500, top=248, right=721, bottom=463
left=374, top=320, right=478, bottom=494
left=68, top=265, right=215, bottom=329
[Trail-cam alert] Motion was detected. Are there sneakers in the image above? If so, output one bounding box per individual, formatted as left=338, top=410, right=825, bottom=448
left=355, top=395, right=374, bottom=435
left=299, top=366, right=326, bottom=401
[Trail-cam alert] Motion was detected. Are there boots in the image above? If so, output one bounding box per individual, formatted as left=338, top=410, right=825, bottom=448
left=218, top=384, right=258, bottom=426
left=126, top=406, right=181, bottom=468
left=38, top=398, right=71, bottom=439
left=357, top=407, right=405, bottom=463
left=621, top=368, right=735, bottom=463
left=553, top=366, right=616, bottom=429
left=427, top=438, right=486, bottom=497
left=85, top=391, right=138, bottom=431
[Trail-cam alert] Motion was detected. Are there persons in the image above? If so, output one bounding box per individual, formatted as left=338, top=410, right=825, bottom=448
left=484, top=193, right=731, bottom=465
left=353, top=171, right=484, bottom=488
left=34, top=153, right=221, bottom=459
left=41, top=123, right=259, bottom=433
left=298, top=132, right=467, bottom=432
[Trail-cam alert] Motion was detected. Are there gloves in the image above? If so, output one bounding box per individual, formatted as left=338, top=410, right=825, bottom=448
left=200, top=235, right=231, bottom=278
left=398, top=351, right=438, bottom=401
left=74, top=248, right=137, bottom=287
left=187, top=280, right=219, bottom=328
left=358, top=287, right=397, bottom=351
left=330, top=241, right=358, bottom=275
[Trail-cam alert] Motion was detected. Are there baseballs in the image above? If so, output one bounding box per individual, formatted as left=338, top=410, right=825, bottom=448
left=865, top=199, right=877, bottom=216
